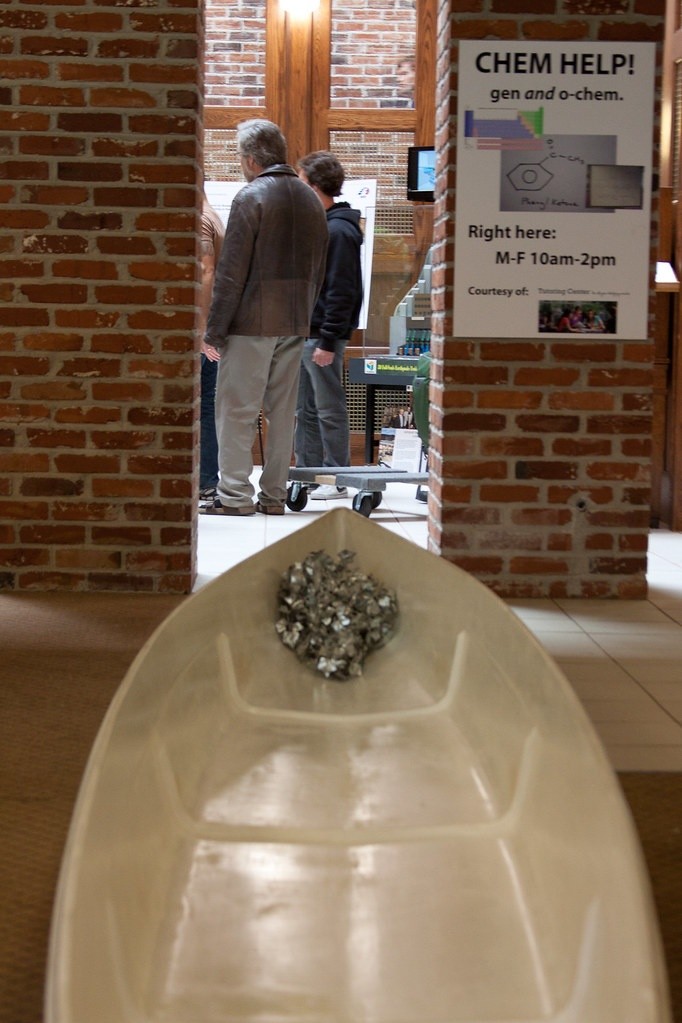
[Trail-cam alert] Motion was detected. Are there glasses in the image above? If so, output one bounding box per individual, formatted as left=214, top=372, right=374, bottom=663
left=234, top=151, right=248, bottom=159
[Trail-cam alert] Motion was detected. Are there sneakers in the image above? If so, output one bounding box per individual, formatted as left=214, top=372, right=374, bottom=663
left=302, top=482, right=318, bottom=493
left=310, top=483, right=348, bottom=500
left=255, top=501, right=285, bottom=514
left=198, top=499, right=256, bottom=515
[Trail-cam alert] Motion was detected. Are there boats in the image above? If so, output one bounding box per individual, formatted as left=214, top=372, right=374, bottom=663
left=38, top=504, right=677, bottom=1023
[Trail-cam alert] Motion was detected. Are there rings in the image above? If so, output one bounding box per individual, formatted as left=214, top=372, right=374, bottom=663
left=208, top=349, right=210, bottom=351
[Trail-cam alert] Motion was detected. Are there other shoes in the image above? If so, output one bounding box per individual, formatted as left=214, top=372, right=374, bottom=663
left=198, top=487, right=219, bottom=501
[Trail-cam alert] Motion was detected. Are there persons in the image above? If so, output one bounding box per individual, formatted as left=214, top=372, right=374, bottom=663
left=539, top=301, right=605, bottom=332
left=389, top=407, right=416, bottom=429
left=199, top=117, right=329, bottom=515
left=293, top=149, right=364, bottom=467
left=199, top=216, right=223, bottom=501
left=396, top=55, right=415, bottom=108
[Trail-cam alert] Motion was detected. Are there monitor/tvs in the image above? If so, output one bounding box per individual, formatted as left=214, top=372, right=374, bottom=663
left=407, top=146, right=434, bottom=202
